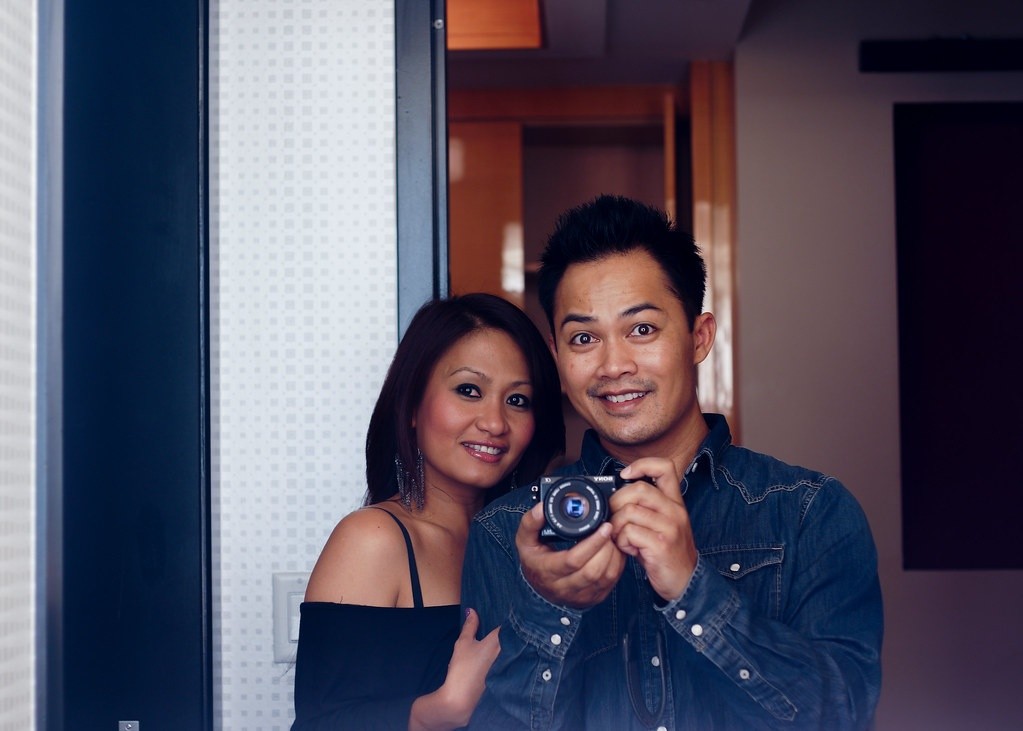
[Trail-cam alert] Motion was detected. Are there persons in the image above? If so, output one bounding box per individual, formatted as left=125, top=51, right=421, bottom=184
left=459, top=193, right=885, bottom=731
left=291, top=293, right=567, bottom=731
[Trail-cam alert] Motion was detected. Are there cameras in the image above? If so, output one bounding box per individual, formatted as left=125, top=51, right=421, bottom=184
left=531, top=472, right=653, bottom=543
